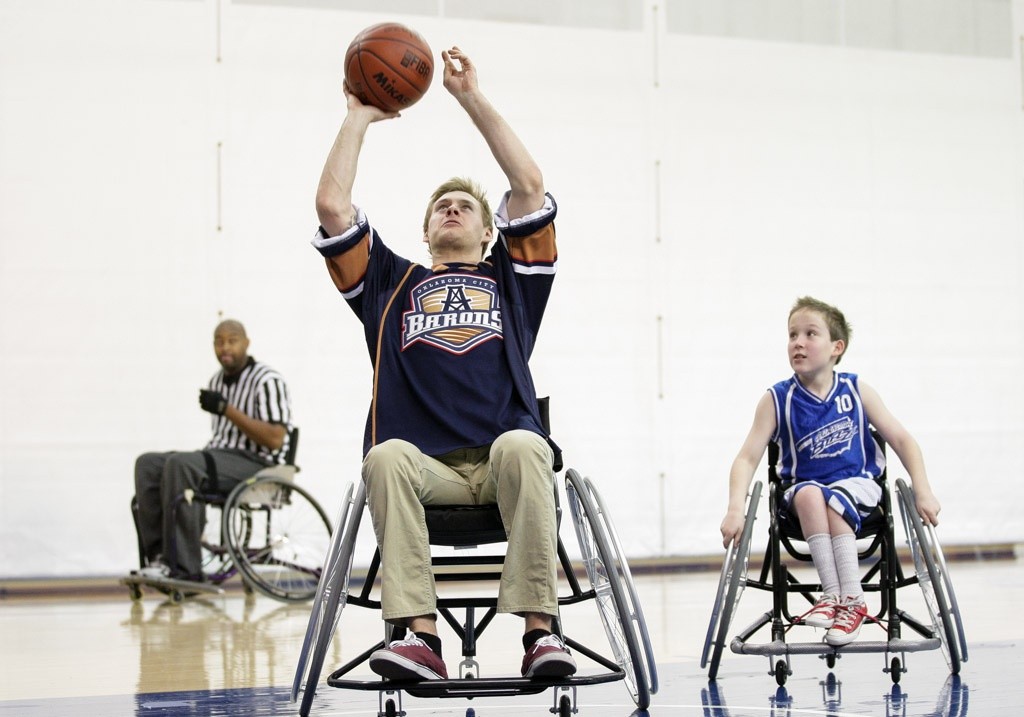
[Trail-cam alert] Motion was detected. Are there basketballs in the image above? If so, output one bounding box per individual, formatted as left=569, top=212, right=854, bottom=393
left=343, top=20, right=435, bottom=111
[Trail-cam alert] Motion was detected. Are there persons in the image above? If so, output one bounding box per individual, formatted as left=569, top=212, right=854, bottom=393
left=719, top=297, right=941, bottom=648
left=134, top=320, right=292, bottom=583
left=313, top=48, right=578, bottom=698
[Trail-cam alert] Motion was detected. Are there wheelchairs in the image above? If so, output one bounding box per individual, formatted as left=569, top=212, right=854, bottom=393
left=293, top=448, right=665, bottom=717
left=119, top=429, right=333, bottom=603
left=702, top=475, right=970, bottom=684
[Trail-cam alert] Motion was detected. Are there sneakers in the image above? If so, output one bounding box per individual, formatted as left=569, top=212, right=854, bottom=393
left=521, top=634, right=577, bottom=695
left=790, top=594, right=843, bottom=628
left=369, top=627, right=448, bottom=681
left=825, top=595, right=880, bottom=646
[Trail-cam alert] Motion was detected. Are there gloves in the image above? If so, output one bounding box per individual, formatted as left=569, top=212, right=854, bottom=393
left=199, top=389, right=227, bottom=416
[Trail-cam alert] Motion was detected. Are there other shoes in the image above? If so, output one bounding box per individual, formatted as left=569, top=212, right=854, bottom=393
left=155, top=571, right=206, bottom=598
left=129, top=564, right=171, bottom=582
